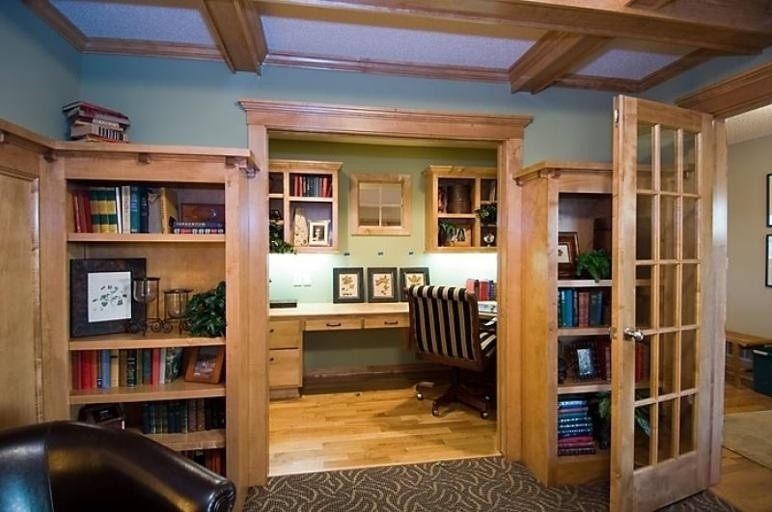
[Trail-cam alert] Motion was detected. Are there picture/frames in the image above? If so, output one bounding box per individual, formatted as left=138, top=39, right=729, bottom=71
left=400, top=267, right=430, bottom=302
left=310, top=222, right=328, bottom=246
left=69, top=257, right=147, bottom=339
left=573, top=346, right=600, bottom=380
left=333, top=267, right=364, bottom=303
left=766, top=173, right=772, bottom=227
left=185, top=345, right=225, bottom=384
left=367, top=267, right=399, bottom=303
left=558, top=232, right=580, bottom=279
left=766, top=234, right=772, bottom=287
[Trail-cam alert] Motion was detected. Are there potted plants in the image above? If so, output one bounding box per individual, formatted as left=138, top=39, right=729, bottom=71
left=574, top=250, right=612, bottom=283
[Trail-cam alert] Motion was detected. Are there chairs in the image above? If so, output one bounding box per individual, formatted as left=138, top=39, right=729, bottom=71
left=408, top=285, right=496, bottom=419
left=0, top=421, right=237, bottom=512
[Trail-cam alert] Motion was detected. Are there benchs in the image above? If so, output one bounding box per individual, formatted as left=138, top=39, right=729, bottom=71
left=725, top=330, right=772, bottom=390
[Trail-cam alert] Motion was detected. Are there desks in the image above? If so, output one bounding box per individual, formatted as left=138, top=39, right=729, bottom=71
left=269, top=303, right=410, bottom=399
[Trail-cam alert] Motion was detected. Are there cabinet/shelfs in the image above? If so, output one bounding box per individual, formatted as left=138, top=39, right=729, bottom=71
left=0, top=121, right=259, bottom=512
left=420, top=164, right=498, bottom=253
left=513, top=161, right=669, bottom=488
left=269, top=159, right=344, bottom=254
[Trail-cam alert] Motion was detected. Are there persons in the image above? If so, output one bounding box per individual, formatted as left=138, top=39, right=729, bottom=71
left=312, top=227, right=324, bottom=241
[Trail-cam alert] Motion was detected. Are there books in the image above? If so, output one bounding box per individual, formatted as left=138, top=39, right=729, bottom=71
left=72, top=181, right=226, bottom=234
left=61, top=98, right=131, bottom=144
left=271, top=298, right=297, bottom=310
left=465, top=277, right=497, bottom=300
left=289, top=171, right=332, bottom=196
left=71, top=346, right=226, bottom=477
left=557, top=286, right=650, bottom=457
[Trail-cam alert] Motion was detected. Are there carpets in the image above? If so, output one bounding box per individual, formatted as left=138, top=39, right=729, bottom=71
left=242, top=455, right=744, bottom=512
left=722, top=409, right=772, bottom=471
